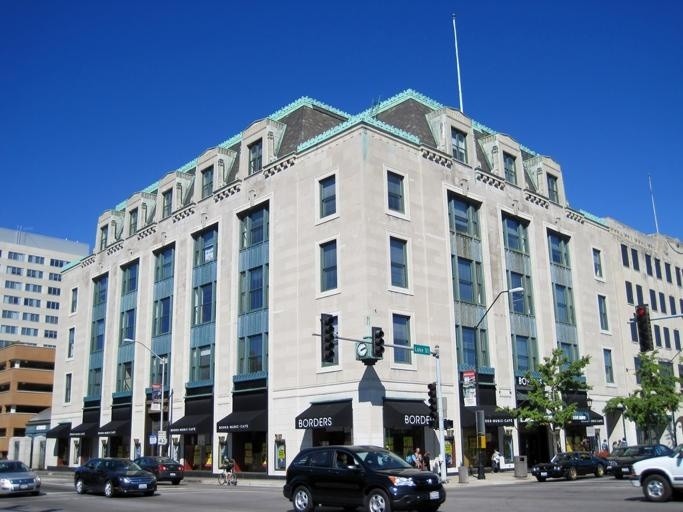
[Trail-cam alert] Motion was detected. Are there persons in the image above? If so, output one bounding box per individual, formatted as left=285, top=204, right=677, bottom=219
left=490, top=448, right=500, bottom=473
left=601, top=439, right=608, bottom=452
left=413, top=447, right=422, bottom=470
left=420, top=451, right=432, bottom=471
left=222, top=455, right=234, bottom=485
left=616, top=440, right=621, bottom=448
left=405, top=449, right=421, bottom=468
left=620, top=437, right=627, bottom=448
left=611, top=441, right=617, bottom=451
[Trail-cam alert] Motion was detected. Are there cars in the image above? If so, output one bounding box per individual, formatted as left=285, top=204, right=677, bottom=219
left=133, top=455, right=184, bottom=486
left=530, top=442, right=682, bottom=504
left=73, top=456, right=156, bottom=498
left=0, top=459, right=41, bottom=497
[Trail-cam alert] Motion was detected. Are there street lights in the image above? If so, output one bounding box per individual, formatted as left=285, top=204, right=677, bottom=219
left=122, top=337, right=169, bottom=457
left=471, top=284, right=524, bottom=480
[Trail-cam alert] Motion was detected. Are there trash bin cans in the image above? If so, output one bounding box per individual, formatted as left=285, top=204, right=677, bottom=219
left=514, top=455, right=527, bottom=478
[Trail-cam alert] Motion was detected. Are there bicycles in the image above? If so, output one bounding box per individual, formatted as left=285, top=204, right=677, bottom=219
left=217, top=455, right=238, bottom=487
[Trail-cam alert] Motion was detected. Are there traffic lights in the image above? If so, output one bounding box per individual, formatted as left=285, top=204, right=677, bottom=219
left=370, top=328, right=385, bottom=359
left=634, top=304, right=654, bottom=355
left=320, top=313, right=339, bottom=365
left=425, top=383, right=437, bottom=419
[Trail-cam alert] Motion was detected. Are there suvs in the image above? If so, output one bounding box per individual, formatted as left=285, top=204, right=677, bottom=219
left=281, top=445, right=447, bottom=512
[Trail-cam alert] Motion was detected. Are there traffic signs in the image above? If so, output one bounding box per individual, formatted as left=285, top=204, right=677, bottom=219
left=412, top=344, right=429, bottom=356
